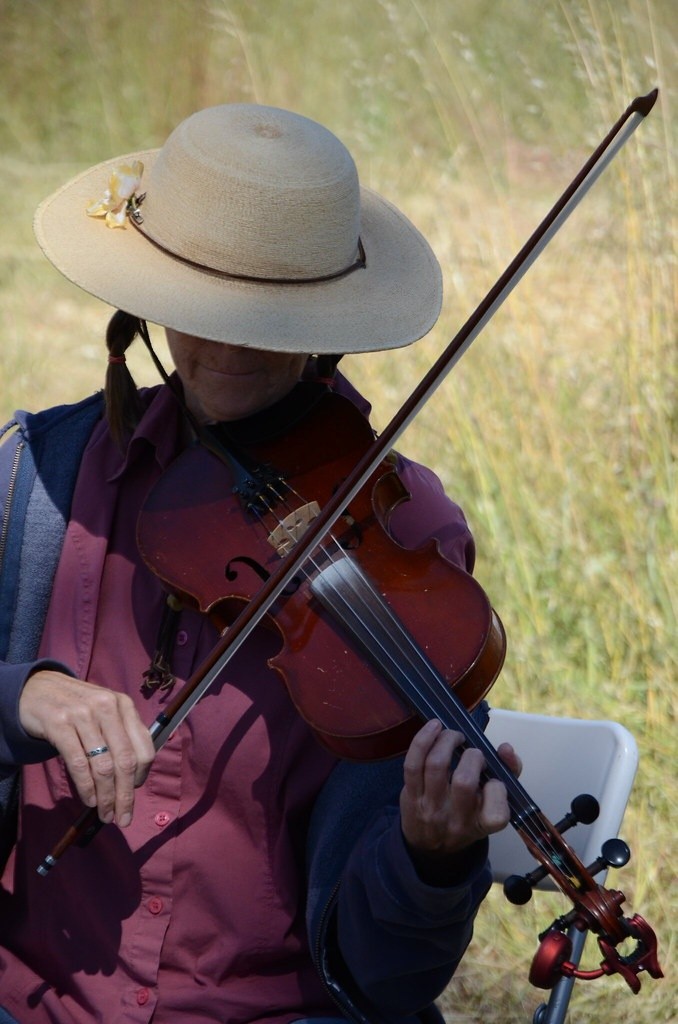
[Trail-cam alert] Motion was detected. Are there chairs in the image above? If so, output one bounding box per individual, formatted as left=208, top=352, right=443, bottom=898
left=483, top=710, right=640, bottom=1024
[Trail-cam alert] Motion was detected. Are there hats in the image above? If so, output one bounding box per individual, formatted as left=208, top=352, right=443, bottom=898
left=32, top=101, right=443, bottom=355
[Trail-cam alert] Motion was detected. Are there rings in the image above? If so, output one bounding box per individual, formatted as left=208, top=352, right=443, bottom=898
left=86, top=746, right=107, bottom=757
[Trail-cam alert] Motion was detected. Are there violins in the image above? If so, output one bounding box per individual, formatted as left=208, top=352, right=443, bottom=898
left=131, top=377, right=663, bottom=996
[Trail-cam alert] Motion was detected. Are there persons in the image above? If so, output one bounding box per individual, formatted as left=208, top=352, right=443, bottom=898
left=0, top=104, right=521, bottom=1024
